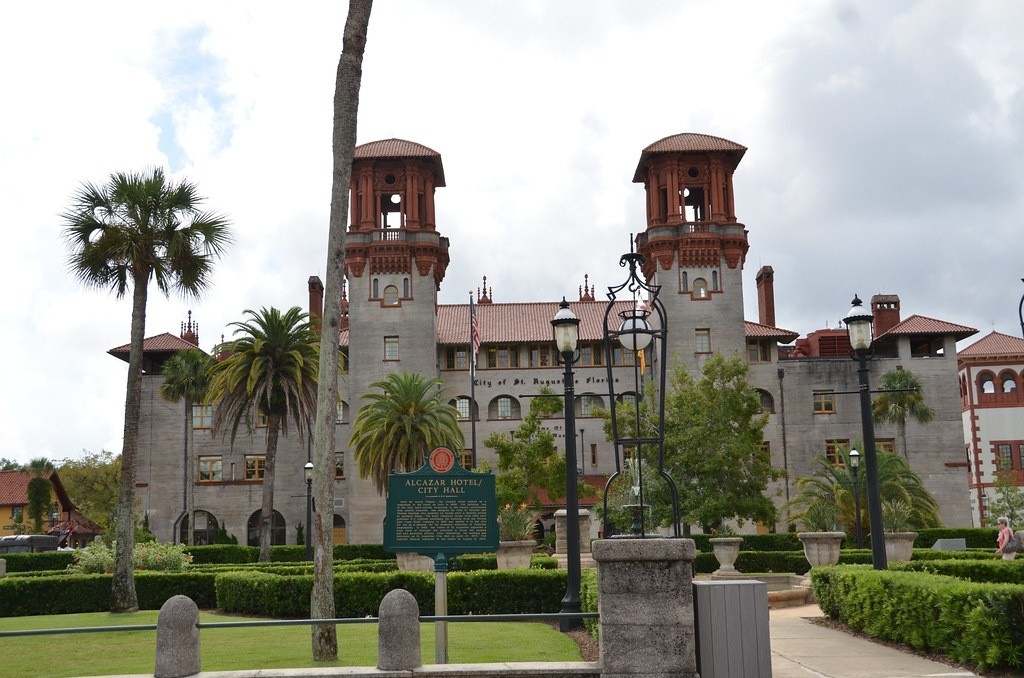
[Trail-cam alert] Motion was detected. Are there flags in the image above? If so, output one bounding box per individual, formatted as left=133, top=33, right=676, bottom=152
left=469, top=302, right=481, bottom=377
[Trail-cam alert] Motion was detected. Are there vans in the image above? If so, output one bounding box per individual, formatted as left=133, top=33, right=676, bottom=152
left=0, top=535, right=58, bottom=554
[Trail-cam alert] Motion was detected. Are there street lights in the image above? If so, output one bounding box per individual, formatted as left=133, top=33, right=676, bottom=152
left=48, top=502, right=60, bottom=536
left=848, top=447, right=863, bottom=550
left=603, top=232, right=681, bottom=540
left=550, top=296, right=584, bottom=632
left=304, top=461, right=314, bottom=561
left=842, top=293, right=888, bottom=571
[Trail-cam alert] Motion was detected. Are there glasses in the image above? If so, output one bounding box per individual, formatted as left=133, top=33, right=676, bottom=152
left=998, top=522, right=1003, bottom=525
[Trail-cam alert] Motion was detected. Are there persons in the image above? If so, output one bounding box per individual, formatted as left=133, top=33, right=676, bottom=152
left=995, top=517, right=1016, bottom=560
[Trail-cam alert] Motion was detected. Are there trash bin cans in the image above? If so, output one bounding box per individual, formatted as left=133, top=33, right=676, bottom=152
left=1, top=533, right=59, bottom=553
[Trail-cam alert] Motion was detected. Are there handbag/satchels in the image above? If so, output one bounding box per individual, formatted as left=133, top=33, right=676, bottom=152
left=1002, top=529, right=1017, bottom=553
